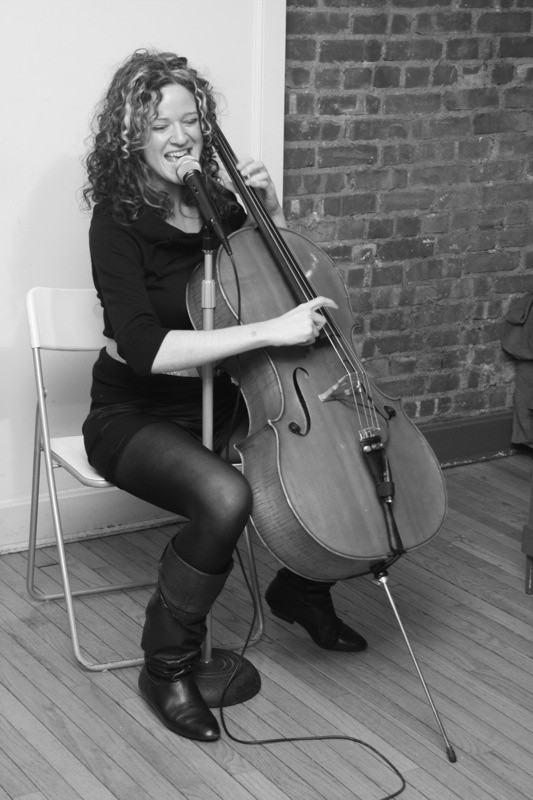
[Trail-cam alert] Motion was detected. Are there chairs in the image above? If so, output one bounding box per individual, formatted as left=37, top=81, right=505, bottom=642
left=25, top=287, right=265, bottom=671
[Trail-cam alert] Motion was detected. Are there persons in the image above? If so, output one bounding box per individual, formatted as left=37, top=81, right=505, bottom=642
left=77, top=50, right=370, bottom=742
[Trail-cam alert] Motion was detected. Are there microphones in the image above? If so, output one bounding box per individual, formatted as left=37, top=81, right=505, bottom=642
left=174, top=155, right=232, bottom=256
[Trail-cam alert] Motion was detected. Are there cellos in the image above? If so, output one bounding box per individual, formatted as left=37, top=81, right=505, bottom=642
left=183, top=119, right=459, bottom=765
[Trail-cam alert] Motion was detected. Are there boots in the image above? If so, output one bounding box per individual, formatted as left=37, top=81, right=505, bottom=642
left=265, top=564, right=373, bottom=654
left=136, top=536, right=236, bottom=742
